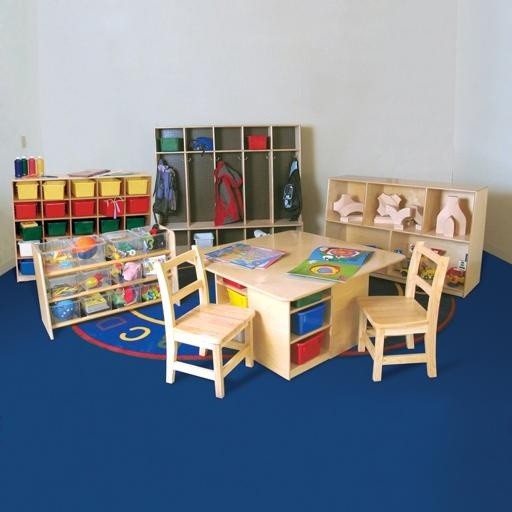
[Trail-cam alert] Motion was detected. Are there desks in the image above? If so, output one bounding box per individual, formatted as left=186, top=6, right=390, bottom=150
left=181, top=228, right=407, bottom=383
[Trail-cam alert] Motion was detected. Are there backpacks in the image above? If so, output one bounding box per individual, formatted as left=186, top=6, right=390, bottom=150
left=281, top=158, right=302, bottom=222
left=153, top=158, right=177, bottom=225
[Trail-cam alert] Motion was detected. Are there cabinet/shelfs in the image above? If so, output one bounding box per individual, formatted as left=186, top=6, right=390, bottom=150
left=9, top=171, right=180, bottom=340
left=320, top=175, right=490, bottom=299
left=154, top=122, right=302, bottom=254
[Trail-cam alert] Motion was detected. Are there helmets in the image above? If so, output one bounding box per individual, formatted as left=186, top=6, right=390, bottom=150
left=191, top=138, right=213, bottom=151
left=52, top=300, right=74, bottom=320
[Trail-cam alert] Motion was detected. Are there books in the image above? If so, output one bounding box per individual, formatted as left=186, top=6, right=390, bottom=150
left=204, top=242, right=283, bottom=269
left=205, top=245, right=288, bottom=268
left=69, top=167, right=135, bottom=179
left=286, top=244, right=375, bottom=284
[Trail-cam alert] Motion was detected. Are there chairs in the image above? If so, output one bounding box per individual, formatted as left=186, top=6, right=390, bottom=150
left=354, top=241, right=451, bottom=383
left=149, top=244, right=257, bottom=399
